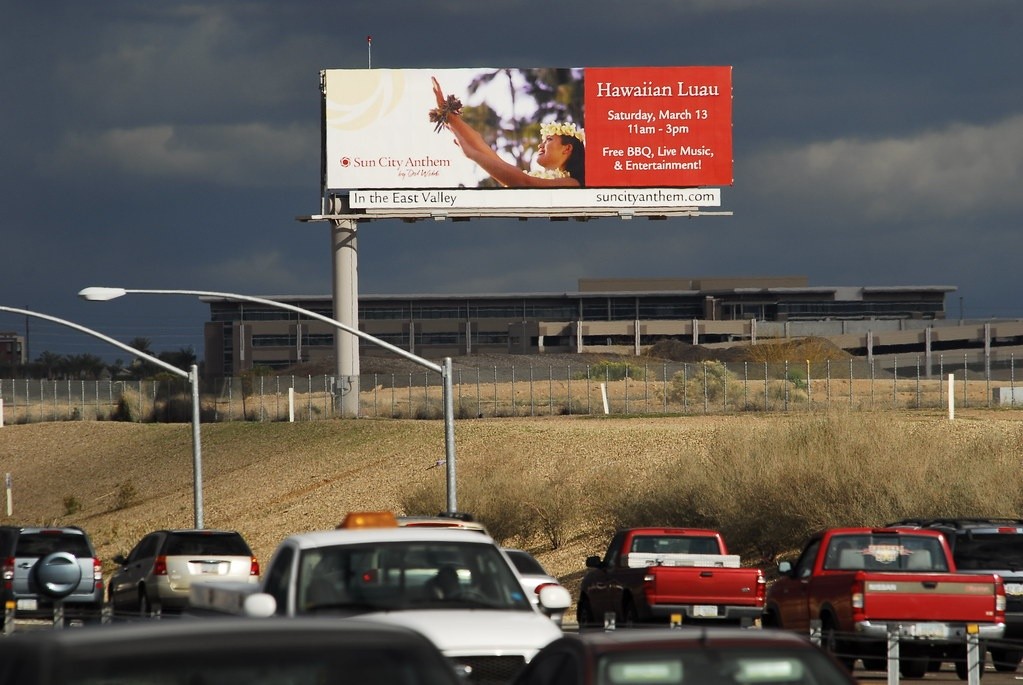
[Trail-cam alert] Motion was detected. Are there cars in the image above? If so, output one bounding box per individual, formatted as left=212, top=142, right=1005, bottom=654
left=500, top=546, right=573, bottom=624
left=262, top=504, right=563, bottom=676
left=533, top=625, right=860, bottom=685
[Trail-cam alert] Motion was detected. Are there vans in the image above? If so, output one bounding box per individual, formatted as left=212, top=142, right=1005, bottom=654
left=0, top=611, right=464, bottom=684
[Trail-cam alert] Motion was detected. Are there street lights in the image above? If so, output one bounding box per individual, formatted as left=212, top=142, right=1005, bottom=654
left=79, top=283, right=461, bottom=514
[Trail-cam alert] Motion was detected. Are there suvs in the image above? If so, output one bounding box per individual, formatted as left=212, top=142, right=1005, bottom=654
left=902, top=518, right=1021, bottom=672
left=1, top=524, right=105, bottom=625
left=106, top=529, right=264, bottom=621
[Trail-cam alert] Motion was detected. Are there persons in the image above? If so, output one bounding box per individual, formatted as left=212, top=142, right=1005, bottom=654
left=828, top=540, right=853, bottom=567
left=426, top=566, right=472, bottom=600
left=425, top=74, right=585, bottom=187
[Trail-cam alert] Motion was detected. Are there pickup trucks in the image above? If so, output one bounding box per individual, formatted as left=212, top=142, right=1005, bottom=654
left=757, top=526, right=1002, bottom=682
left=575, top=525, right=764, bottom=631
